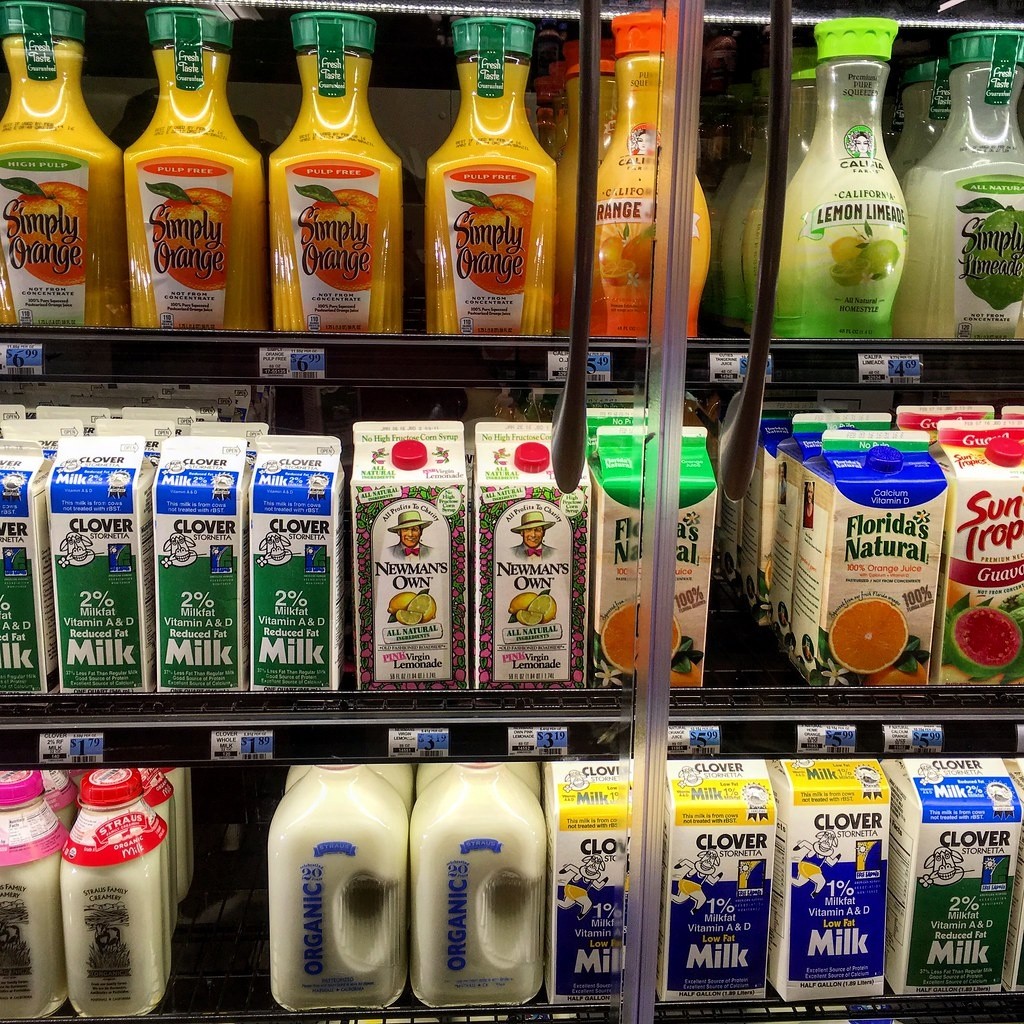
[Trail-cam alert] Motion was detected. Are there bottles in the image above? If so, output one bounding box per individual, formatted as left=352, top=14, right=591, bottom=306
left=0, top=759, right=545, bottom=1018
left=0, top=0, right=1024, bottom=347
